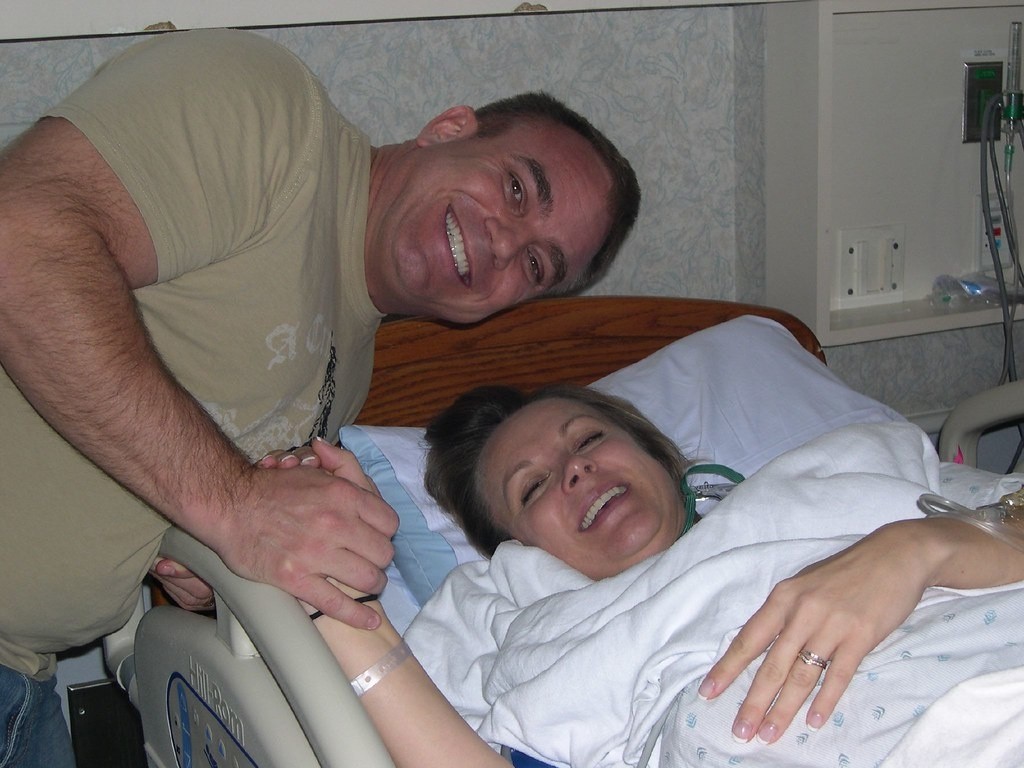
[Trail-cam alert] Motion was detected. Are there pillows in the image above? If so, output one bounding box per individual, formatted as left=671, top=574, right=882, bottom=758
left=336, top=315, right=910, bottom=609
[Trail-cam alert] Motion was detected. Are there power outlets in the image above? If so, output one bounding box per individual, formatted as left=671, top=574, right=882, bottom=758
left=837, top=222, right=906, bottom=298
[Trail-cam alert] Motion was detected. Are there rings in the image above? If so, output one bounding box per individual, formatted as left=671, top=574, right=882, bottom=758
left=797, top=649, right=827, bottom=669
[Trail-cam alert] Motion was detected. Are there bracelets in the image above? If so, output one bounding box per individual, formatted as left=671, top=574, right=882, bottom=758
left=309, top=594, right=378, bottom=621
left=349, top=638, right=413, bottom=698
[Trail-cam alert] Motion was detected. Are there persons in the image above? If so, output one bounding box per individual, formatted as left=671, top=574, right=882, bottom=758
left=257, top=383, right=1024, bottom=768
left=0, top=27, right=642, bottom=768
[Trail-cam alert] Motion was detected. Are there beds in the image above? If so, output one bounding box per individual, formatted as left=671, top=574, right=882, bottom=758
left=97, top=298, right=1024, bottom=768
